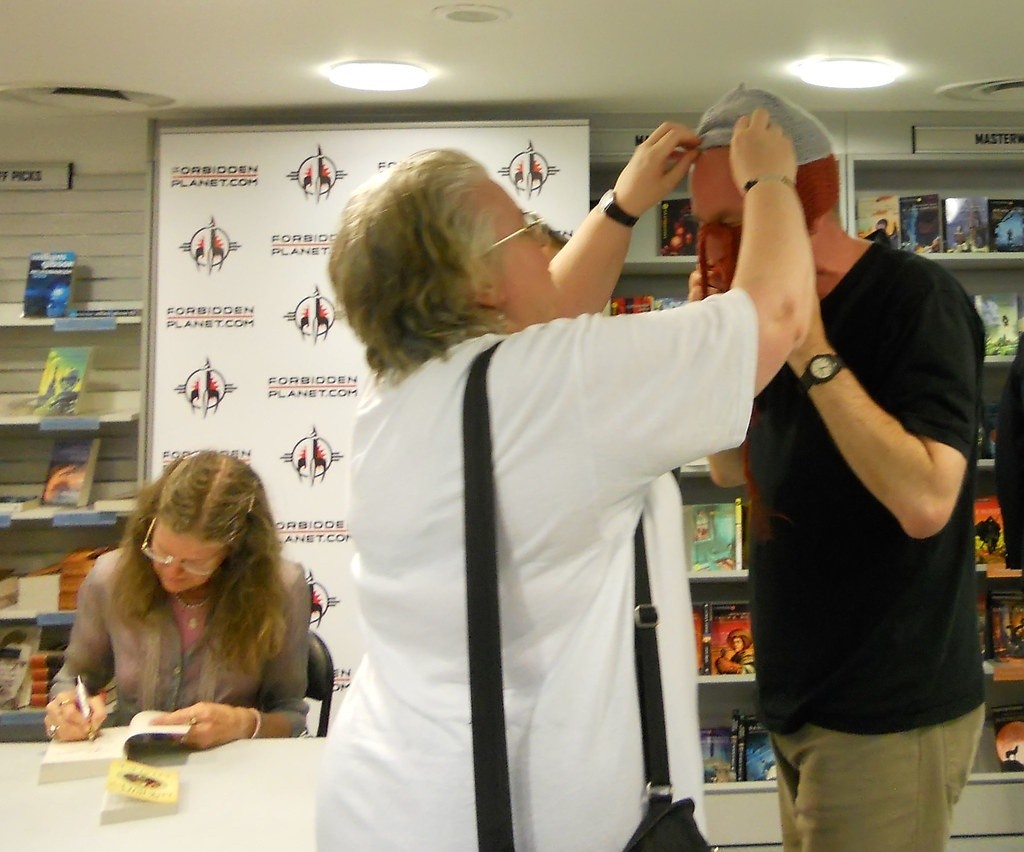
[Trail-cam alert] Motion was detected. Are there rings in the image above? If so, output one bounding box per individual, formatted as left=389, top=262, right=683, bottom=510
left=48, top=734, right=55, bottom=740
left=50, top=725, right=61, bottom=734
left=190, top=717, right=198, bottom=724
left=59, top=699, right=71, bottom=707
left=180, top=735, right=188, bottom=744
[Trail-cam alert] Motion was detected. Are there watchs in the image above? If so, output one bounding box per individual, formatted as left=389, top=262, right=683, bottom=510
left=599, top=188, right=639, bottom=228
left=799, top=354, right=847, bottom=391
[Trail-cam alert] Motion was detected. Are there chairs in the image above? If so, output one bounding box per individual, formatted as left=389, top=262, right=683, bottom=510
left=305, top=631, right=334, bottom=737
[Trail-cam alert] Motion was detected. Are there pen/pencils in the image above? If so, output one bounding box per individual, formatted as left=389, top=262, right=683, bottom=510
left=76, top=674, right=95, bottom=742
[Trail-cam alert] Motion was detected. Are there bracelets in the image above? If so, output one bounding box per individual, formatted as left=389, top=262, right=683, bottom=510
left=248, top=707, right=262, bottom=739
left=742, top=175, right=798, bottom=194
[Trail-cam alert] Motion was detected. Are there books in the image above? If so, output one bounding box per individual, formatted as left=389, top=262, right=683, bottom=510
left=856, top=193, right=1024, bottom=361
left=974, top=398, right=1024, bottom=772
left=680, top=498, right=780, bottom=784
left=40, top=435, right=102, bottom=509
left=21, top=251, right=76, bottom=319
left=30, top=344, right=92, bottom=418
left=0, top=543, right=119, bottom=709
left=606, top=193, right=696, bottom=317
left=99, top=760, right=178, bottom=826
left=37, top=709, right=191, bottom=782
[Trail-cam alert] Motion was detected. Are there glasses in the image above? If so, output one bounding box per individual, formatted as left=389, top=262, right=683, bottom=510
left=140, top=518, right=222, bottom=577
left=474, top=212, right=550, bottom=258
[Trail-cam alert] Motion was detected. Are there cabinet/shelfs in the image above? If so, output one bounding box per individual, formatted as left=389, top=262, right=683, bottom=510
left=598, top=253, right=1024, bottom=852
left=0, top=302, right=144, bottom=744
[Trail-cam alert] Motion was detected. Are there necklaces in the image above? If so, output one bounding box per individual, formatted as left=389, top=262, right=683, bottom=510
left=175, top=592, right=211, bottom=607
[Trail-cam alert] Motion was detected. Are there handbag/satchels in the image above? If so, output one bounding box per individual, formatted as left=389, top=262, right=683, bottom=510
left=623, top=796, right=713, bottom=852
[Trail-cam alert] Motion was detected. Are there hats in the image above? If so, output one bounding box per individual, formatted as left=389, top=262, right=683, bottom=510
left=692, top=82, right=831, bottom=168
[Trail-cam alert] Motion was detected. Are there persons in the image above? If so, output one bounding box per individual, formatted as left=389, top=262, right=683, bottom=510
left=43, top=447, right=310, bottom=750
left=317, top=105, right=816, bottom=852
left=687, top=79, right=983, bottom=852
left=0, top=631, right=31, bottom=708
